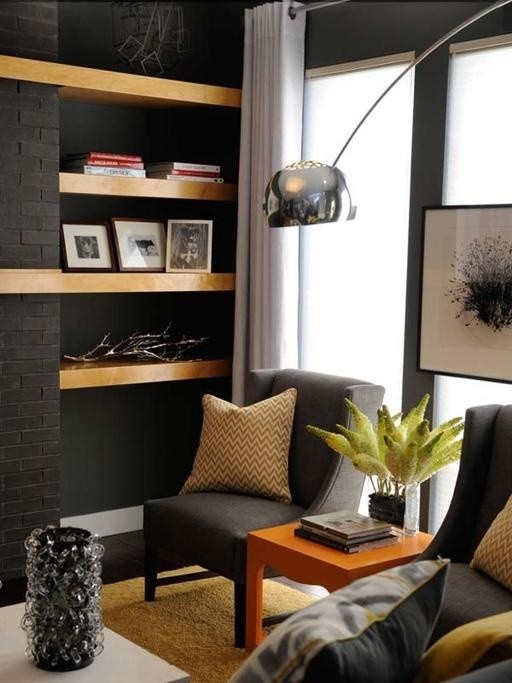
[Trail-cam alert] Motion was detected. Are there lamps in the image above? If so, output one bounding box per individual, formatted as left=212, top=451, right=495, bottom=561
left=262, top=0, right=512, bottom=228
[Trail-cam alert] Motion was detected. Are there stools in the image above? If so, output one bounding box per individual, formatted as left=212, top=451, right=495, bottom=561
left=0, top=601, right=190, bottom=683
left=420, top=609, right=512, bottom=683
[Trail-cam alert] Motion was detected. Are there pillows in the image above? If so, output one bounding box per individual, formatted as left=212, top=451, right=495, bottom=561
left=180, top=387, right=298, bottom=501
left=226, top=558, right=450, bottom=683
left=470, top=493, right=512, bottom=589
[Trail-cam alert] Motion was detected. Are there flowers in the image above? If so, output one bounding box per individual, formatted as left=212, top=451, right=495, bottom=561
left=304, top=393, right=466, bottom=496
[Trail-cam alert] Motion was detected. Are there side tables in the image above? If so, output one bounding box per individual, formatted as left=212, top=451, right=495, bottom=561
left=246, top=510, right=435, bottom=652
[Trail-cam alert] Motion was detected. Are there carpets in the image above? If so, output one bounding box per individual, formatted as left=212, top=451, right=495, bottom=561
left=100, top=565, right=320, bottom=683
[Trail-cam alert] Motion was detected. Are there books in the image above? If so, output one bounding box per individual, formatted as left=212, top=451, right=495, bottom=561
left=294, top=509, right=400, bottom=554
left=60, top=150, right=224, bottom=183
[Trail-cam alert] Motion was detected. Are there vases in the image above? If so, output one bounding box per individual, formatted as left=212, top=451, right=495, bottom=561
left=368, top=492, right=406, bottom=527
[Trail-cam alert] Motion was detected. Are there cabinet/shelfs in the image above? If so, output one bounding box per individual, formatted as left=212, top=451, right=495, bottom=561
left=57, top=62, right=241, bottom=585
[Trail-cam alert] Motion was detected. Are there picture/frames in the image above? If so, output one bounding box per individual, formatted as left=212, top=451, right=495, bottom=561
left=110, top=216, right=164, bottom=272
left=60, top=222, right=115, bottom=272
left=165, top=220, right=212, bottom=274
left=416, top=204, right=512, bottom=384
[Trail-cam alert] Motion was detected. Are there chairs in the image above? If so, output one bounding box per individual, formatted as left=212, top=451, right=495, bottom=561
left=142, top=368, right=385, bottom=644
left=418, top=405, right=512, bottom=660
